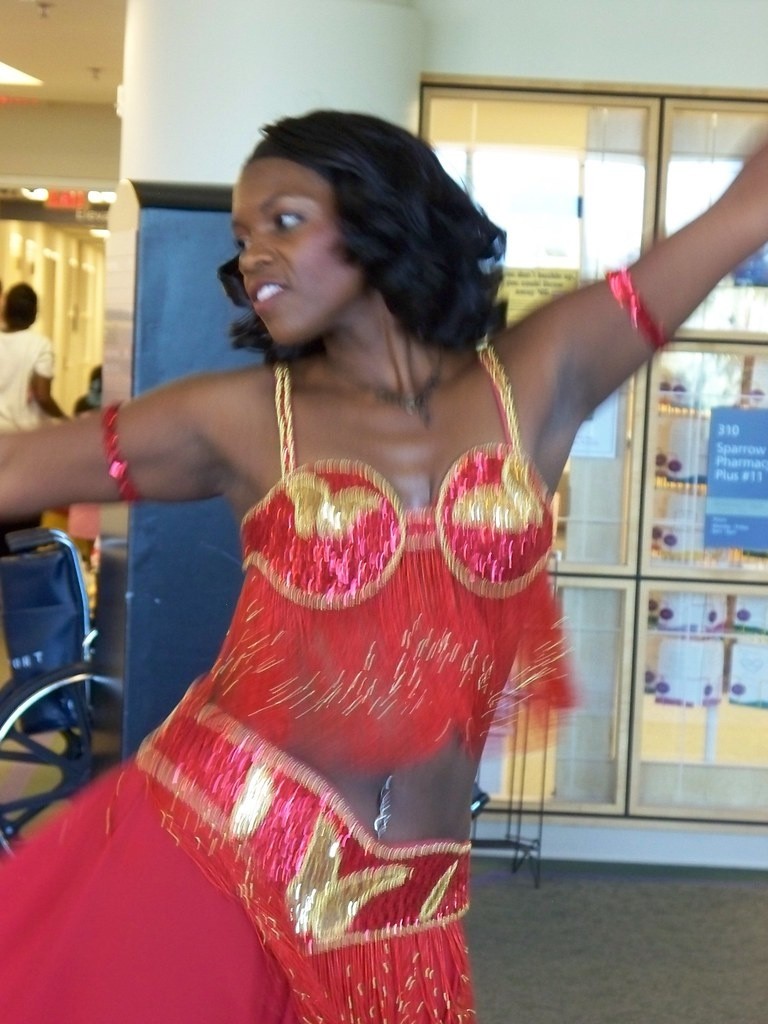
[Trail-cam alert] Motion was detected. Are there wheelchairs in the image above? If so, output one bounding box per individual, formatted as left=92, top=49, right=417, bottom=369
left=0, top=521, right=136, bottom=846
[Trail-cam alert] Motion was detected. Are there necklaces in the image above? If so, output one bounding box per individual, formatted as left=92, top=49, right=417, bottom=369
left=373, top=362, right=445, bottom=431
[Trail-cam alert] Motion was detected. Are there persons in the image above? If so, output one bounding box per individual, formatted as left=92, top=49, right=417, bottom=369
left=0, top=281, right=64, bottom=554
left=0, top=109, right=767, bottom=1024
left=70, top=367, right=103, bottom=568
